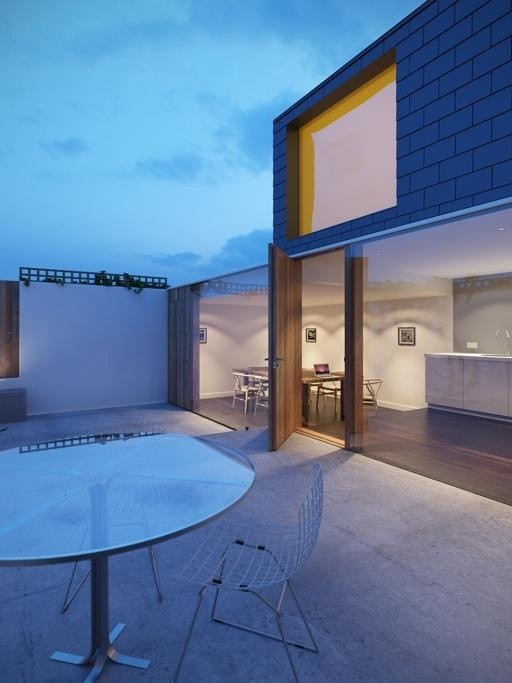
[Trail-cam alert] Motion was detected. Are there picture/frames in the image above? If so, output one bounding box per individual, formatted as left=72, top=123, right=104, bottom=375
left=199, top=327, right=207, bottom=343
left=306, top=327, right=316, bottom=342
left=397, top=326, right=415, bottom=346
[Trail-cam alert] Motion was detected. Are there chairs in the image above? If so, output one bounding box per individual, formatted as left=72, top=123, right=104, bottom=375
left=232, top=364, right=383, bottom=415
left=173, top=460, right=325, bottom=682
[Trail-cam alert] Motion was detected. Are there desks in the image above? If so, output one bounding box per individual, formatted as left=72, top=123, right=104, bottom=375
left=232, top=367, right=345, bottom=426
left=0, top=430, right=255, bottom=682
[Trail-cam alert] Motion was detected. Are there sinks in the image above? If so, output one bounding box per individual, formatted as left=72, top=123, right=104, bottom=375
left=482, top=355, right=511, bottom=357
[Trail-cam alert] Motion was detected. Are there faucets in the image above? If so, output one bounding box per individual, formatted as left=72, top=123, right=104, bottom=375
left=495, top=326, right=511, bottom=356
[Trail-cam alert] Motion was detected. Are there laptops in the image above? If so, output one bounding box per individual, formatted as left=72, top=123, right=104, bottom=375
left=313, top=363, right=331, bottom=377
